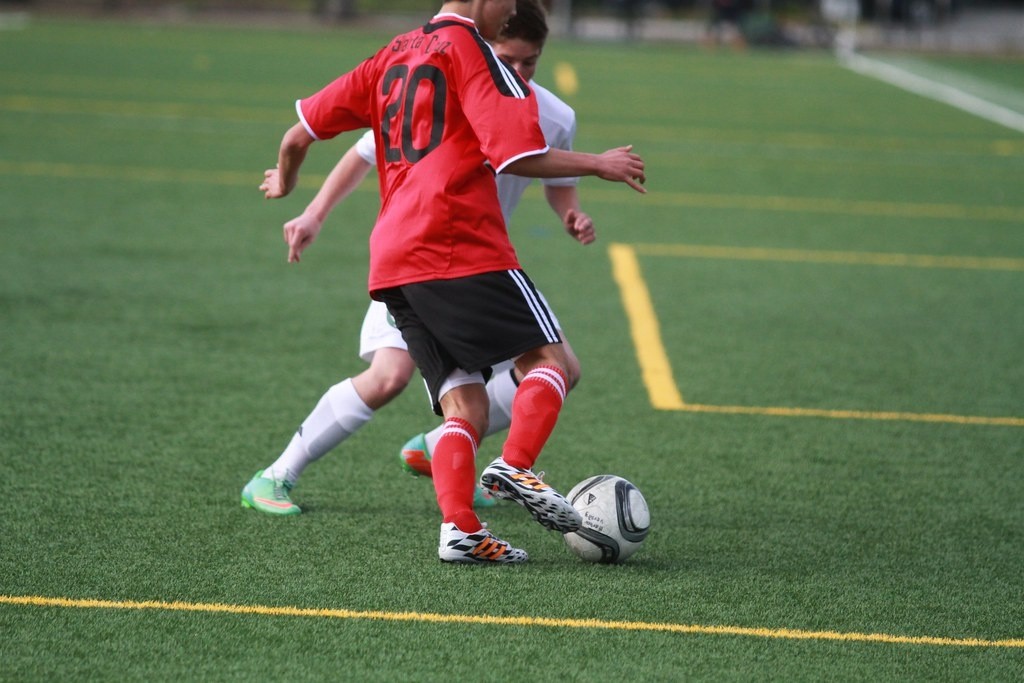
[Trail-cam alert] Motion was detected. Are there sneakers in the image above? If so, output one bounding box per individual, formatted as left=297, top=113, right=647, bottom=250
left=400, top=432, right=496, bottom=507
left=438, top=514, right=529, bottom=563
left=479, top=457, right=583, bottom=534
left=241, top=470, right=302, bottom=515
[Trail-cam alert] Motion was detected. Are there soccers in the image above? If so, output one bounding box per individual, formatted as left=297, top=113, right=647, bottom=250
left=563, top=474, right=650, bottom=563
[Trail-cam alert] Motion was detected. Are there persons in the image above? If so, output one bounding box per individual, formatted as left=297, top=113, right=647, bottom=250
left=240, top=0, right=596, bottom=514
left=258, top=0, right=646, bottom=563
